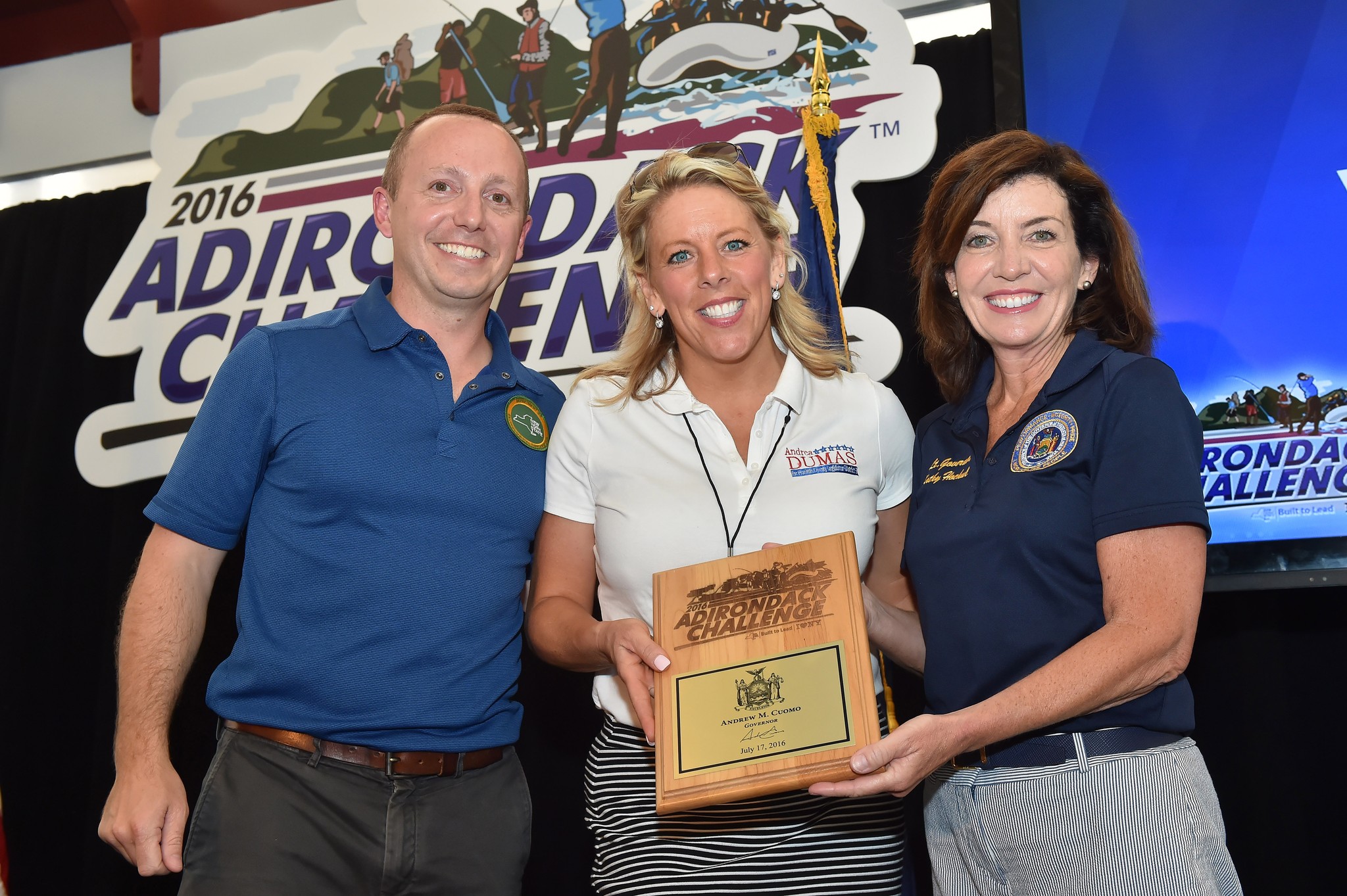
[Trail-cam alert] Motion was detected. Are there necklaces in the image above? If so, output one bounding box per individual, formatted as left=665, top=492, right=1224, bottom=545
left=684, top=407, right=790, bottom=556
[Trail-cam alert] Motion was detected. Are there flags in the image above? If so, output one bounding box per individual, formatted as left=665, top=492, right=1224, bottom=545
left=799, top=108, right=853, bottom=375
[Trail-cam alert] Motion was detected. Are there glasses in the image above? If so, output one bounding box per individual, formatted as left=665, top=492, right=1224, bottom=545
left=630, top=141, right=761, bottom=198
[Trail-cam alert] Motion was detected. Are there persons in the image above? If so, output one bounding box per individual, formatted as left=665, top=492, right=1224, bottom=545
left=525, top=139, right=915, bottom=896
left=98, top=104, right=566, bottom=896
left=810, top=129, right=1244, bottom=896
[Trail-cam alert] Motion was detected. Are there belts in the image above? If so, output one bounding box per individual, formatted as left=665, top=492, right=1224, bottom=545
left=223, top=713, right=514, bottom=780
left=941, top=726, right=1185, bottom=770
